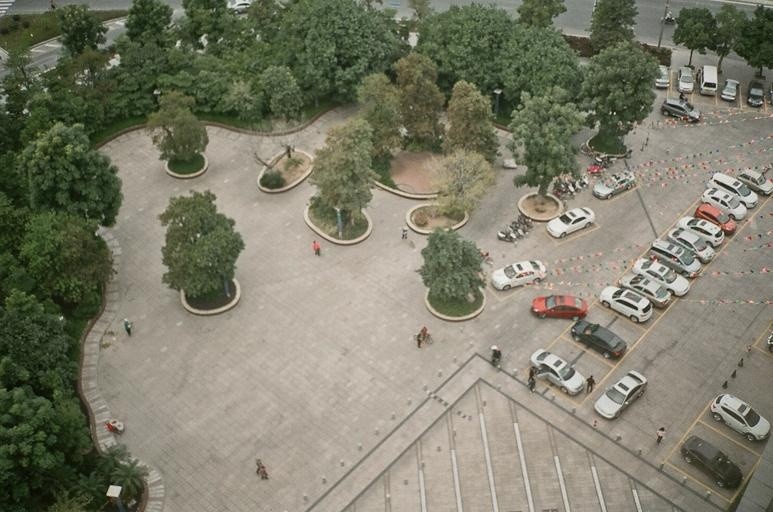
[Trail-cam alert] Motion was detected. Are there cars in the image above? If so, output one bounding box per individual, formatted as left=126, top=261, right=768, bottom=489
left=225, top=0, right=253, bottom=16
left=530, top=349, right=590, bottom=399
left=617, top=270, right=673, bottom=311
left=592, top=168, right=639, bottom=203
left=629, top=259, right=692, bottom=299
left=651, top=61, right=773, bottom=124
left=489, top=257, right=550, bottom=291
left=707, top=393, right=773, bottom=446
left=767, top=332, right=773, bottom=355
left=593, top=367, right=650, bottom=424
left=676, top=434, right=750, bottom=495
left=568, top=316, right=629, bottom=362
left=530, top=291, right=590, bottom=321
left=545, top=207, right=600, bottom=242
left=649, top=167, right=773, bottom=280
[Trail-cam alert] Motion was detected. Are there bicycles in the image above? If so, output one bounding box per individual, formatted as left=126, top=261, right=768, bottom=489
left=413, top=333, right=437, bottom=346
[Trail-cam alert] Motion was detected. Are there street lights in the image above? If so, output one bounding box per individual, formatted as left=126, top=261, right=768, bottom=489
left=106, top=484, right=125, bottom=512
left=492, top=87, right=503, bottom=117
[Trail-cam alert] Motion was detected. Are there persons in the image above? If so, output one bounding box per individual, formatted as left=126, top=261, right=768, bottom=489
left=106, top=422, right=120, bottom=435
left=528, top=366, right=536, bottom=382
left=124, top=318, right=133, bottom=337
left=656, top=426, right=665, bottom=445
left=587, top=375, right=595, bottom=393
left=416, top=334, right=422, bottom=349
left=313, top=240, right=321, bottom=256
left=491, top=344, right=501, bottom=369
left=421, top=327, right=428, bottom=341
left=679, top=92, right=685, bottom=101
left=665, top=11, right=673, bottom=22
left=256, top=458, right=269, bottom=479
left=401, top=223, right=409, bottom=239
left=527, top=379, right=535, bottom=393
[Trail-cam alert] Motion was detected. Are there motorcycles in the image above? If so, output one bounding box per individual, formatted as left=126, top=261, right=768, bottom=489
left=548, top=143, right=616, bottom=203
left=659, top=15, right=677, bottom=26
left=469, top=249, right=496, bottom=283
left=497, top=212, right=536, bottom=246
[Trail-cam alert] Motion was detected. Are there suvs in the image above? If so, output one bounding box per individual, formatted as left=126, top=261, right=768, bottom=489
left=598, top=285, right=656, bottom=328
